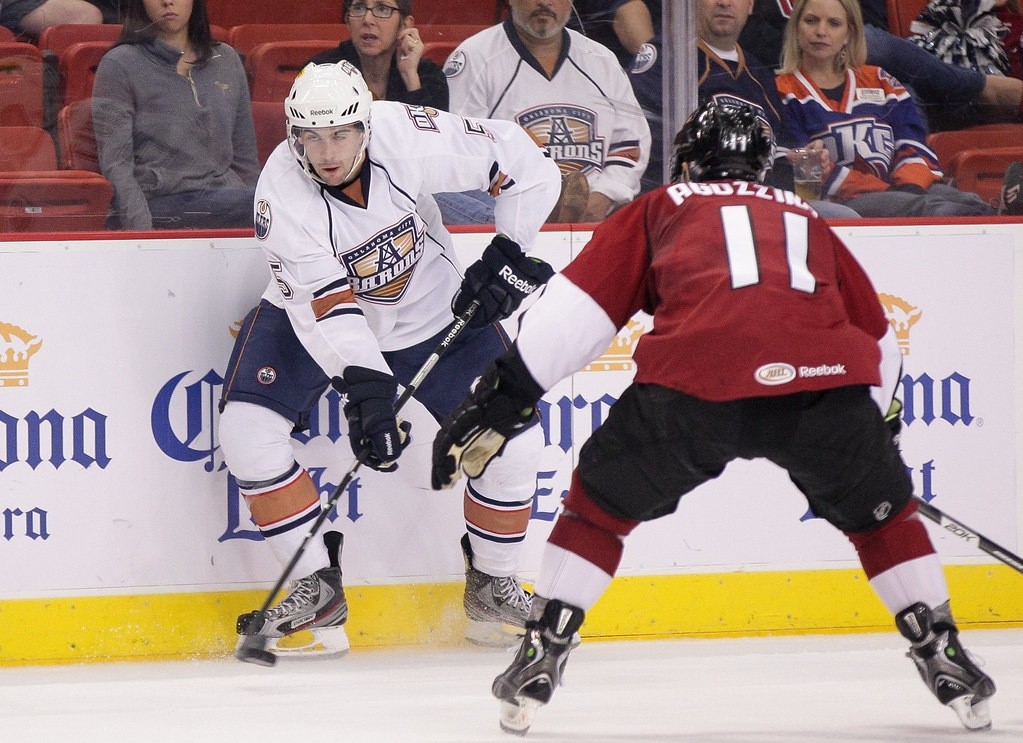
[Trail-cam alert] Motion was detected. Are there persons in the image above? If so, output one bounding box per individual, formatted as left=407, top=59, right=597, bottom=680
left=432, top=101, right=996, bottom=710
left=219, top=58, right=562, bottom=636
left=442, top=0, right=1022, bottom=224
left=0, top=0, right=260, bottom=232
left=301, top=0, right=449, bottom=111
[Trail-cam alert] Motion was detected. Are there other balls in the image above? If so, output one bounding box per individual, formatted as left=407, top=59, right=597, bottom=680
left=243, top=647, right=277, bottom=667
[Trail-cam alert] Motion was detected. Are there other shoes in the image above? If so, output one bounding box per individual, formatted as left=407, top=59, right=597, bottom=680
left=991, top=162, right=1023, bottom=215
left=553, top=172, right=589, bottom=222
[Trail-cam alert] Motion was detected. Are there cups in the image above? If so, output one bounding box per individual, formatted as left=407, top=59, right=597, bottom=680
left=790, top=146, right=825, bottom=205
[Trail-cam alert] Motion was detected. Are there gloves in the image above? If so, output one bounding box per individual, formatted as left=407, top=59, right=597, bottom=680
left=451, top=235, right=555, bottom=328
left=432, top=358, right=541, bottom=489
left=332, top=367, right=401, bottom=472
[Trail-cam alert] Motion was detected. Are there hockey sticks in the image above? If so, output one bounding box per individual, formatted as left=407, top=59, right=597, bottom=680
left=912, top=494, right=1023, bottom=575
left=236, top=298, right=480, bottom=663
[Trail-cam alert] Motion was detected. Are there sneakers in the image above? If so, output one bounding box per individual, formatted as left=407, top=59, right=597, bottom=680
left=236, top=531, right=349, bottom=657
left=492, top=599, right=584, bottom=732
left=895, top=602, right=996, bottom=729
left=461, top=533, right=581, bottom=648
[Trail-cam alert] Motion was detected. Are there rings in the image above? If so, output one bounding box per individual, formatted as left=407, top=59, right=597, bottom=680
left=401, top=54, right=410, bottom=60
left=413, top=38, right=418, bottom=43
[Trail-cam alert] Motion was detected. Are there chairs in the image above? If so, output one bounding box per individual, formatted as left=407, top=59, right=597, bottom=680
left=0, top=0, right=1023, bottom=226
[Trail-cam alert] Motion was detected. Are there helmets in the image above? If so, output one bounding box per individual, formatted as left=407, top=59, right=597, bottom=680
left=284, top=60, right=373, bottom=147
left=668, top=94, right=775, bottom=184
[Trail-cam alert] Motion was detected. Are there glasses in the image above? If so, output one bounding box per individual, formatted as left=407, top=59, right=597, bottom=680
left=348, top=4, right=400, bottom=18
